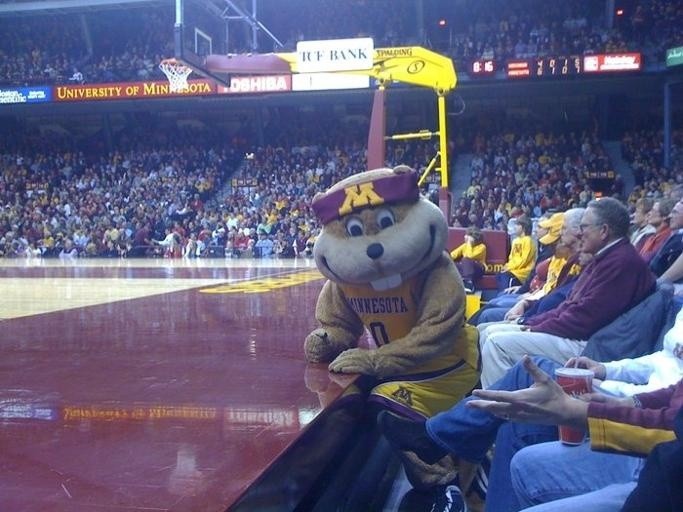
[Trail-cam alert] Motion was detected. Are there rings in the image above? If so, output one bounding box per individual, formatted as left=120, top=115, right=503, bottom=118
left=504, top=414, right=509, bottom=420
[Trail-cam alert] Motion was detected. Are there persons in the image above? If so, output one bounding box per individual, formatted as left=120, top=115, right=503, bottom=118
left=0, top=0, right=681, bottom=90
left=450, top=227, right=488, bottom=296
left=1, top=112, right=682, bottom=260
left=378, top=178, right=683, bottom=512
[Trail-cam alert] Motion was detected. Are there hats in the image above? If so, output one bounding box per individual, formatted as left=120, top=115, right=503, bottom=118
left=512, top=213, right=531, bottom=225
left=537, top=214, right=565, bottom=245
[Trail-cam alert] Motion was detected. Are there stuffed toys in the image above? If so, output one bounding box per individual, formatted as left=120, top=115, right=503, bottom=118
left=304, top=165, right=480, bottom=511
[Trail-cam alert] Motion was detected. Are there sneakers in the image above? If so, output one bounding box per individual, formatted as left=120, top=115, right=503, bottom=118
left=378, top=411, right=448, bottom=464
left=431, top=485, right=469, bottom=511
left=465, top=281, right=474, bottom=293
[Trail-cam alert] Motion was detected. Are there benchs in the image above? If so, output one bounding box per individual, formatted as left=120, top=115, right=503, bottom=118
left=444, top=227, right=511, bottom=290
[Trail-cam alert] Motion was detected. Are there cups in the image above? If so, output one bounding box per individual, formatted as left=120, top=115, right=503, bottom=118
left=555, top=367, right=594, bottom=446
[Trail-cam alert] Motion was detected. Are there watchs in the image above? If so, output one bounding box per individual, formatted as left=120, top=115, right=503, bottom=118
left=518, top=319, right=524, bottom=325
